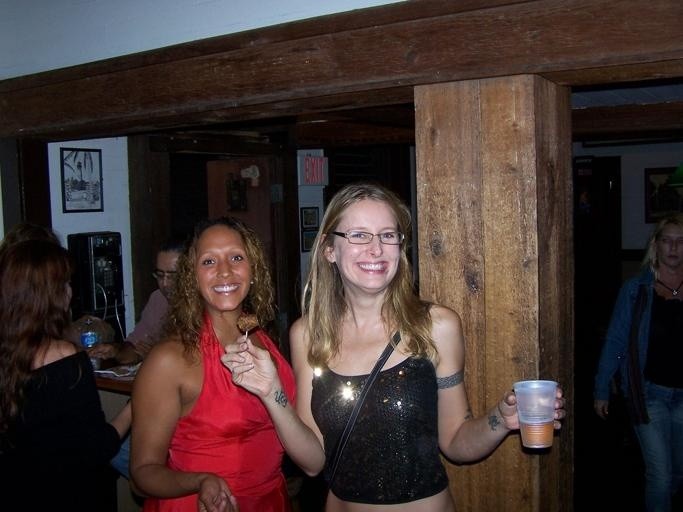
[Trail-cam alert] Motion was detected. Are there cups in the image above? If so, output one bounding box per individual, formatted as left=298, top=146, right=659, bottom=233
left=514, top=380, right=558, bottom=451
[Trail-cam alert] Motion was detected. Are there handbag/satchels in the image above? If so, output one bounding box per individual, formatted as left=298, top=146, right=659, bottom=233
left=109, top=434, right=131, bottom=479
left=614, top=358, right=650, bottom=424
left=300, top=471, right=331, bottom=512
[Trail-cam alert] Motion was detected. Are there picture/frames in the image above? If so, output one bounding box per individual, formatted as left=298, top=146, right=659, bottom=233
left=60, top=146, right=105, bottom=213
left=300, top=206, right=319, bottom=252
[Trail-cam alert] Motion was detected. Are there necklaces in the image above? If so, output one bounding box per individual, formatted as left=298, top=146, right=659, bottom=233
left=655, top=277, right=683, bottom=296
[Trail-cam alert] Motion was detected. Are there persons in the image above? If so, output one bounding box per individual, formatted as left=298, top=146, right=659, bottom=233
left=88, top=240, right=188, bottom=374
left=130, top=216, right=297, bottom=512
left=0, top=241, right=133, bottom=512
left=219, top=184, right=567, bottom=512
left=594, top=214, right=683, bottom=512
left=0, top=224, right=58, bottom=250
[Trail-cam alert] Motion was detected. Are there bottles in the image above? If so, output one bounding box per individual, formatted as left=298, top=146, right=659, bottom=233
left=80, top=317, right=101, bottom=369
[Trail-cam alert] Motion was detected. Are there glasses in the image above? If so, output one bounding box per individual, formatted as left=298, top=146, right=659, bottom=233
left=333, top=231, right=405, bottom=245
left=151, top=271, right=178, bottom=279
